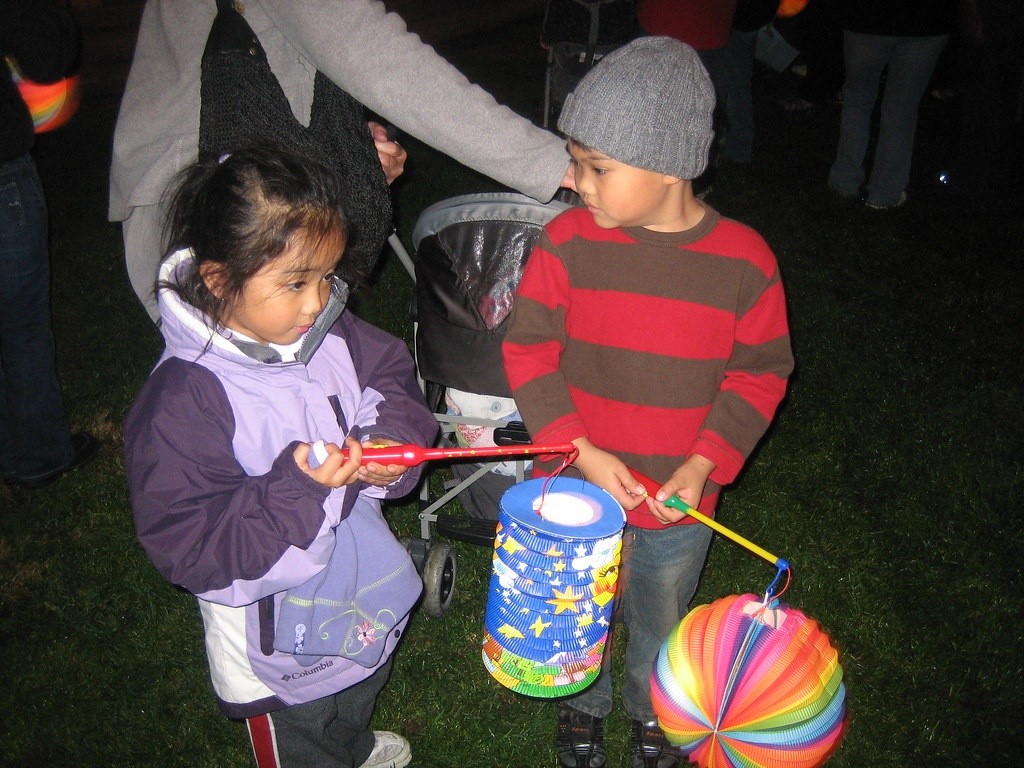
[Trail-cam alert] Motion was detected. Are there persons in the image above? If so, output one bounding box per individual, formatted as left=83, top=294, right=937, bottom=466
left=500, top=51, right=794, bottom=768
left=1, top=1, right=102, bottom=495
left=108, top=0, right=580, bottom=355
left=538, top=0, right=965, bottom=209
left=126, top=143, right=441, bottom=766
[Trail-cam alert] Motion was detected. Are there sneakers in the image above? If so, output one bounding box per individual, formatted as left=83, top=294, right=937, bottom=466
left=556, top=711, right=606, bottom=768
left=358, top=731, right=412, bottom=768
left=630, top=719, right=681, bottom=768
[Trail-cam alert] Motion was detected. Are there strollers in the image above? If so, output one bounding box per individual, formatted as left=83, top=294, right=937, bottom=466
left=387, top=43, right=624, bottom=618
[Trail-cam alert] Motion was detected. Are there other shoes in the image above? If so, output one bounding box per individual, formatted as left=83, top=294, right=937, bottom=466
left=828, top=163, right=859, bottom=200
left=865, top=191, right=907, bottom=211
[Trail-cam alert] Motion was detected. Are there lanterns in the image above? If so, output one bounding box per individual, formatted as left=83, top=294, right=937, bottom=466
left=480, top=476, right=628, bottom=700
left=648, top=595, right=848, bottom=767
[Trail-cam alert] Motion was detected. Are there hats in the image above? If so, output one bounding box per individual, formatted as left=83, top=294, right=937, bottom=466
left=558, top=35, right=716, bottom=179
